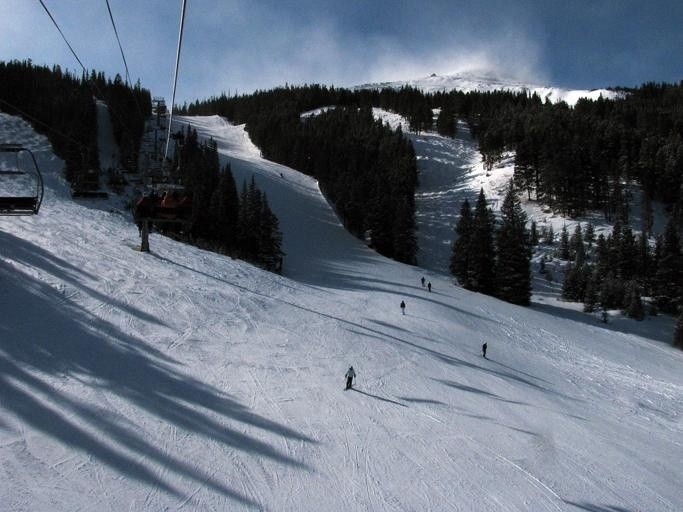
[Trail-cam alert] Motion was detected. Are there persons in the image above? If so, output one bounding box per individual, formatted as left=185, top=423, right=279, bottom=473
left=344, top=364, right=357, bottom=390
left=420, top=276, right=425, bottom=287
left=481, top=342, right=488, bottom=357
left=399, top=300, right=405, bottom=314
left=427, top=281, right=431, bottom=291
left=279, top=172, right=283, bottom=178
